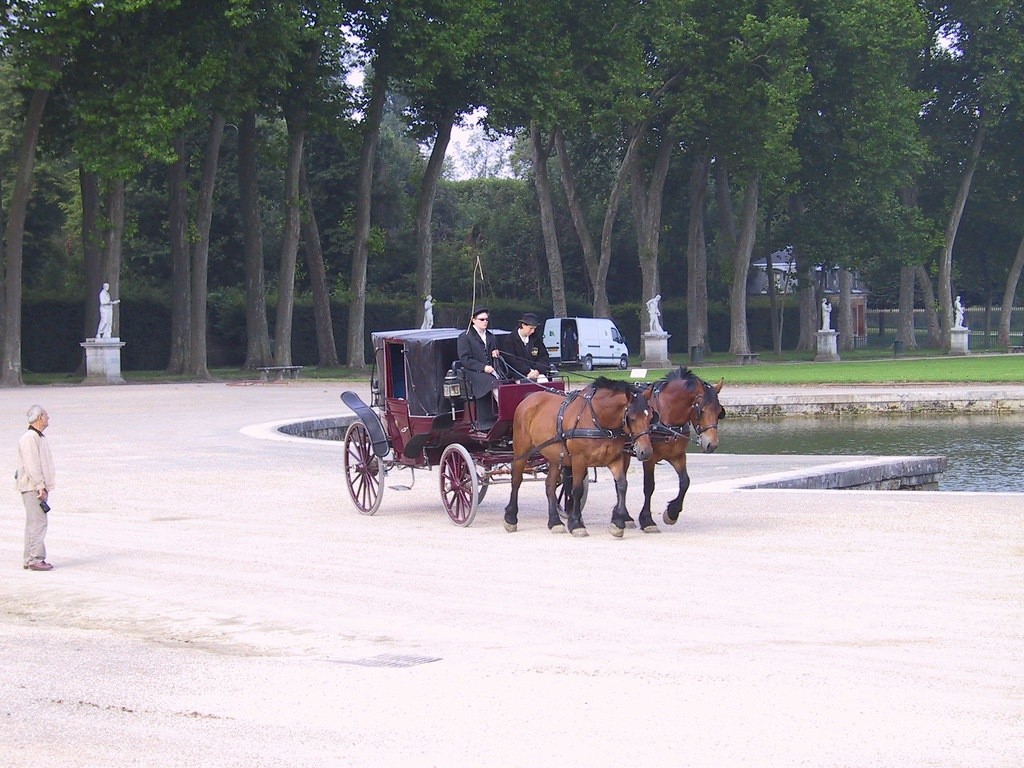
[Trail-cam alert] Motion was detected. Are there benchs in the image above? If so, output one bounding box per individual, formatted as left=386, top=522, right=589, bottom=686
left=736, top=354, right=759, bottom=365
left=256, top=365, right=303, bottom=379
left=451, top=361, right=474, bottom=401
left=1008, top=346, right=1023, bottom=353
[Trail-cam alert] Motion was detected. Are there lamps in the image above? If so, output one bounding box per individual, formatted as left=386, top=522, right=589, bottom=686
left=443, top=370, right=461, bottom=421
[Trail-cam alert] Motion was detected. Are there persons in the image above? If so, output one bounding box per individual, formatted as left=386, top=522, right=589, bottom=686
left=499, top=312, right=551, bottom=380
left=646, top=295, right=664, bottom=333
left=457, top=305, right=502, bottom=432
left=822, top=298, right=832, bottom=330
left=96, top=283, right=121, bottom=338
left=424, top=295, right=434, bottom=329
left=954, top=295, right=964, bottom=327
left=563, top=327, right=577, bottom=360
left=17, top=405, right=55, bottom=570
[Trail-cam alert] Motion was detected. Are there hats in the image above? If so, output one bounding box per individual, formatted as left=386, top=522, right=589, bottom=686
left=473, top=304, right=490, bottom=315
left=518, top=313, right=541, bottom=327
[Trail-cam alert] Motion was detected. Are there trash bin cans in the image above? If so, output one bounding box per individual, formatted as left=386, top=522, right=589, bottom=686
left=894, top=340, right=903, bottom=354
left=691, top=346, right=703, bottom=364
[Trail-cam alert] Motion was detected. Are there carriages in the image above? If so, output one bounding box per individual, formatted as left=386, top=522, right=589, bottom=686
left=340, top=325, right=725, bottom=538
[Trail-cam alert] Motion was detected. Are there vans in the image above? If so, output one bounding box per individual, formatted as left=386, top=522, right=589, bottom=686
left=542, top=317, right=628, bottom=372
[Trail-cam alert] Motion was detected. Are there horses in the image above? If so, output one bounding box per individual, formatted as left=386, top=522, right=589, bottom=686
left=503, top=376, right=655, bottom=538
left=613, top=364, right=724, bottom=534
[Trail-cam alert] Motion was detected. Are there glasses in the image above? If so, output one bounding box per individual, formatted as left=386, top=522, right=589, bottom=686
left=475, top=317, right=490, bottom=322
